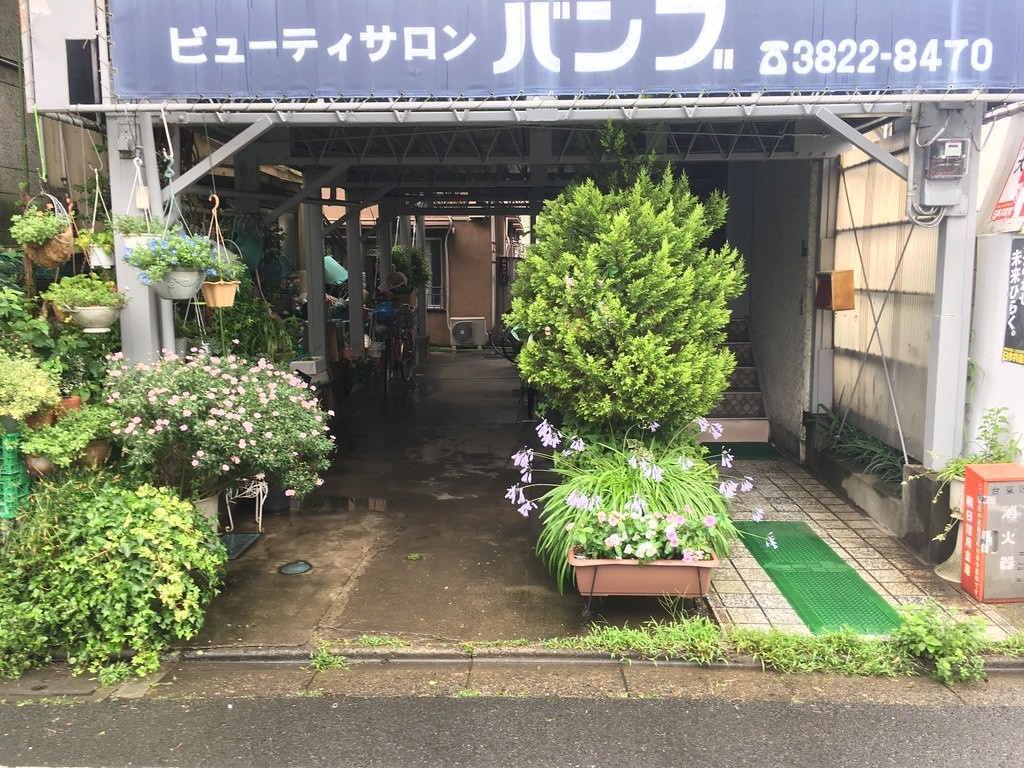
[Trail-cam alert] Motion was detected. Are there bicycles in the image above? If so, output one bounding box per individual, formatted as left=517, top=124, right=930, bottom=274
left=490, top=307, right=544, bottom=425
left=370, top=304, right=416, bottom=400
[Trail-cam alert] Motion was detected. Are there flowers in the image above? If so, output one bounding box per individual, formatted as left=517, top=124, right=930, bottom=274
left=100, top=339, right=340, bottom=501
left=8, top=181, right=75, bottom=246
left=504, top=415, right=778, bottom=597
left=122, top=225, right=249, bottom=287
left=501, top=116, right=751, bottom=442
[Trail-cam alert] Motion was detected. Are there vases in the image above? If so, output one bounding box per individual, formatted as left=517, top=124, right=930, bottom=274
left=290, top=356, right=327, bottom=374
left=147, top=267, right=207, bottom=299
left=568, top=545, right=719, bottom=598
left=193, top=494, right=219, bottom=535
left=200, top=281, right=241, bottom=308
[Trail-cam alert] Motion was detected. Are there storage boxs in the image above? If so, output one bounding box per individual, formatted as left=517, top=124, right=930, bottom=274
left=0, top=433, right=37, bottom=521
left=393, top=287, right=416, bottom=309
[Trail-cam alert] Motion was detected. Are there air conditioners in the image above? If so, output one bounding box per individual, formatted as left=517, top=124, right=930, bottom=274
left=449, top=317, right=487, bottom=351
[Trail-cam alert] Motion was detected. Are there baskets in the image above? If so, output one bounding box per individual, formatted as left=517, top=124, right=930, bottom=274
left=22, top=193, right=74, bottom=270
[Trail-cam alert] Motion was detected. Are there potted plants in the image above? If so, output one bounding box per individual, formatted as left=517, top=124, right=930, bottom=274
left=74, top=227, right=115, bottom=266
left=38, top=272, right=134, bottom=334
left=103, top=212, right=178, bottom=257
left=900, top=404, right=1024, bottom=541
left=0, top=244, right=120, bottom=478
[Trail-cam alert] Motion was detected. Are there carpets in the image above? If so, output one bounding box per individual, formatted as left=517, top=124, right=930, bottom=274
left=702, top=443, right=785, bottom=460
